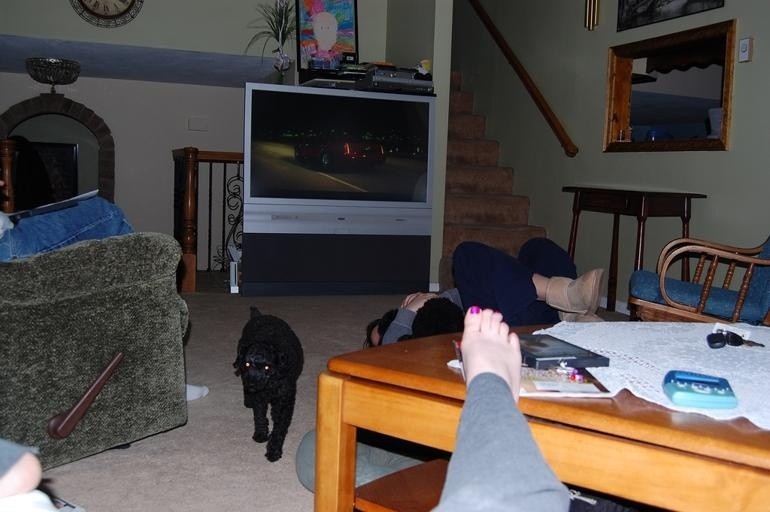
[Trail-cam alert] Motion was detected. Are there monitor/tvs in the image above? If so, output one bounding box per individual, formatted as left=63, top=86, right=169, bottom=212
left=240, top=82, right=437, bottom=236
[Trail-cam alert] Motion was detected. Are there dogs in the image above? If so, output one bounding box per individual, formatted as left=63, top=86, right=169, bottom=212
left=232, top=306, right=304, bottom=463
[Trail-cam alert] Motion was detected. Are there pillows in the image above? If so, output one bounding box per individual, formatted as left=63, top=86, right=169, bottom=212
left=295, top=427, right=427, bottom=493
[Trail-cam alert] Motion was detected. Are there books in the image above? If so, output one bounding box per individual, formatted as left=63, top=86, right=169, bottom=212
left=663, top=370, right=737, bottom=410
left=452, top=336, right=616, bottom=399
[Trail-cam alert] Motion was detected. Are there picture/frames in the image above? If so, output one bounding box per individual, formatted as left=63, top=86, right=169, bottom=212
left=295, top=0, right=359, bottom=72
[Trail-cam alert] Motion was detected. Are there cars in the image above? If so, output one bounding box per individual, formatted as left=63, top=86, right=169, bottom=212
left=292, top=124, right=388, bottom=174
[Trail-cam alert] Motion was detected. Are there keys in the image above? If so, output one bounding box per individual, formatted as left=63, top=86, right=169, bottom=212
left=707, top=329, right=765, bottom=349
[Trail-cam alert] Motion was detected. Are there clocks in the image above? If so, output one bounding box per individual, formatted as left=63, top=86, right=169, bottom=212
left=70, top=0, right=144, bottom=29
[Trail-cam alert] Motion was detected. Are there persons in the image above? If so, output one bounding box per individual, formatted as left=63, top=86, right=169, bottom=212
left=2, top=440, right=45, bottom=497
left=437, top=306, right=572, bottom=510
left=364, top=237, right=605, bottom=348
left=0, top=195, right=133, bottom=263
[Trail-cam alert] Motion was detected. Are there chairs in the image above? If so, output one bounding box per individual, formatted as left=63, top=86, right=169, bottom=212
left=0, top=200, right=190, bottom=470
left=627, top=232, right=770, bottom=325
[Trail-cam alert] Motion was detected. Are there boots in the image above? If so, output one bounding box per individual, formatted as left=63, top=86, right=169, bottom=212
left=545, top=268, right=607, bottom=321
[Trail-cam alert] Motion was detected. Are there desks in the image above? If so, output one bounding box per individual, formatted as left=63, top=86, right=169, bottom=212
left=561, top=182, right=706, bottom=310
left=318, top=320, right=770, bottom=512
